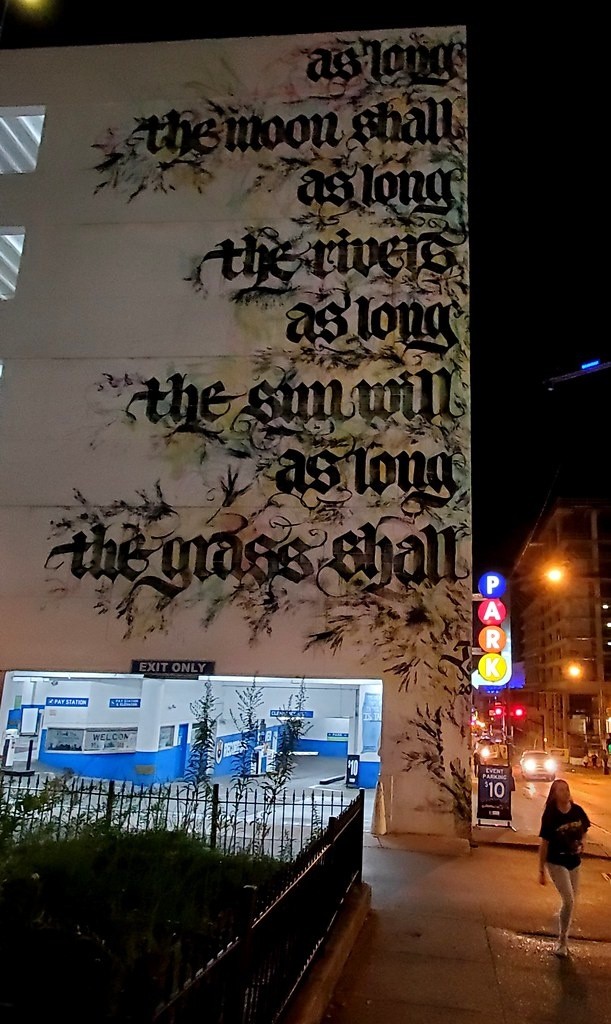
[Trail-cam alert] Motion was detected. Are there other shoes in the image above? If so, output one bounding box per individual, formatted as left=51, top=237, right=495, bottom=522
left=554, top=940, right=568, bottom=958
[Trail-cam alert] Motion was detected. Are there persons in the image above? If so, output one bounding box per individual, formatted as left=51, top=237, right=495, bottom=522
left=538, top=779, right=591, bottom=957
left=589, top=751, right=599, bottom=768
left=582, top=751, right=591, bottom=768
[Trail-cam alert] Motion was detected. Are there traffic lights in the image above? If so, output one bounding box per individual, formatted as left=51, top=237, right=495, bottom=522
left=494, top=706, right=504, bottom=717
left=514, top=707, right=525, bottom=720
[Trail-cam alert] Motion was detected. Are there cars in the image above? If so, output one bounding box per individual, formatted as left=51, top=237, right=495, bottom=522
left=519, top=750, right=556, bottom=782
